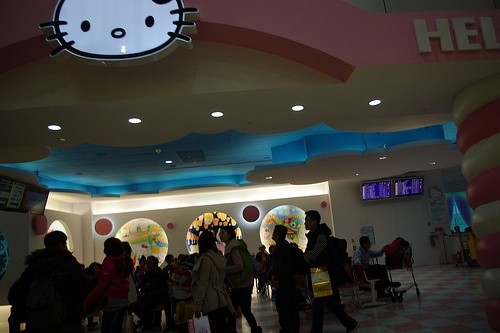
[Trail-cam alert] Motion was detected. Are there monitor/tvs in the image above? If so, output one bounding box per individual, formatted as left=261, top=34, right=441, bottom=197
left=393, top=177, right=424, bottom=200
left=360, top=179, right=393, bottom=201
left=0, top=176, right=49, bottom=215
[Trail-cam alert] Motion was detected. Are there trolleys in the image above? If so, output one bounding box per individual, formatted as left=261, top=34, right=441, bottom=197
left=384, top=243, right=421, bottom=302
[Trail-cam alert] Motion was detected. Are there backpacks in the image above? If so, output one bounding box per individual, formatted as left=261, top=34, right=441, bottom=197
left=24, top=264, right=67, bottom=331
left=320, top=232, right=348, bottom=269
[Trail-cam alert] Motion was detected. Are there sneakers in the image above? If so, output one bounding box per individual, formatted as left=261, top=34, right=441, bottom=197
left=250, top=317, right=359, bottom=333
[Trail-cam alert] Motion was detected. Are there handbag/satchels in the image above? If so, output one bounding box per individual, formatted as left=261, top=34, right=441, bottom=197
left=174, top=300, right=195, bottom=326
left=121, top=310, right=135, bottom=333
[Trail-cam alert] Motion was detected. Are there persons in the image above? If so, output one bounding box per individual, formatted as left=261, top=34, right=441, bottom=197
left=191, top=230, right=237, bottom=333
left=77, top=252, right=204, bottom=332
left=305, top=209, right=361, bottom=333
left=271, top=224, right=304, bottom=333
left=219, top=225, right=264, bottom=333
left=254, top=243, right=310, bottom=298
left=9, top=231, right=91, bottom=332
left=120, top=241, right=139, bottom=333
left=84, top=238, right=131, bottom=333
left=352, top=236, right=402, bottom=297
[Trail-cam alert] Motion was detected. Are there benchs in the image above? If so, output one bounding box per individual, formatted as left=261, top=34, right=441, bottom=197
left=352, top=263, right=386, bottom=308
